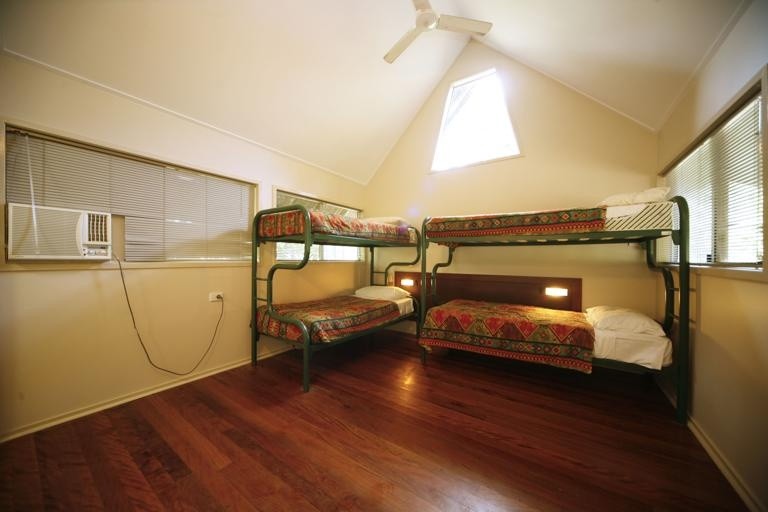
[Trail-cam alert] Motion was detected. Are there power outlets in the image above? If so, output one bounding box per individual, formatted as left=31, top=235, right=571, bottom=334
left=209, top=292, right=224, bottom=301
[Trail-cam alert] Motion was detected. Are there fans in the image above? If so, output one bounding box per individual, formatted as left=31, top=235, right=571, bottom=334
left=382, top=0, right=494, bottom=64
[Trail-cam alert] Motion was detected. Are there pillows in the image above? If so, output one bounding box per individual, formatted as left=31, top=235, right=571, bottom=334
left=585, top=305, right=667, bottom=338
left=362, top=216, right=410, bottom=226
left=354, top=285, right=411, bottom=300
left=596, top=186, right=671, bottom=204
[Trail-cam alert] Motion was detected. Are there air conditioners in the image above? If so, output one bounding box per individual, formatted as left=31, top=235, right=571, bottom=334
left=7, top=202, right=111, bottom=261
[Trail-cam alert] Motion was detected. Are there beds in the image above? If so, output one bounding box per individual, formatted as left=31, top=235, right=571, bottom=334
left=250, top=203, right=423, bottom=391
left=419, top=191, right=689, bottom=427
